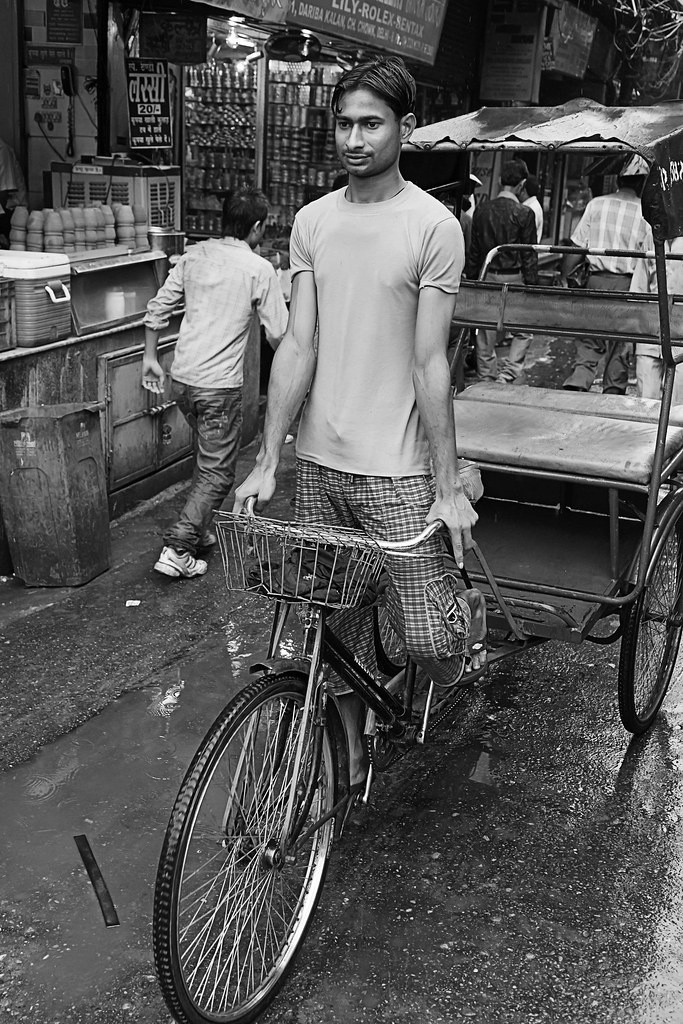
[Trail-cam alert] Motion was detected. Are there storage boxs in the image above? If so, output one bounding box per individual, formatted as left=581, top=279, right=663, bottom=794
left=0, top=277, right=18, bottom=353
left=0, top=249, right=73, bottom=348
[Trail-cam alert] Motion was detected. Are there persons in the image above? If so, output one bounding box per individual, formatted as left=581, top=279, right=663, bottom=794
left=461, top=160, right=683, bottom=406
left=233, top=57, right=486, bottom=822
left=141, top=184, right=290, bottom=578
left=0, top=138, right=29, bottom=251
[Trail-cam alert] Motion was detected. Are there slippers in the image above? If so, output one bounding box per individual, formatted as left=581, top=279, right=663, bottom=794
left=298, top=771, right=377, bottom=822
left=453, top=588, right=488, bottom=687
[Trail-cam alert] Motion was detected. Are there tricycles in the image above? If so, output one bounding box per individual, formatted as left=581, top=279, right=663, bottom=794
left=151, top=101, right=682, bottom=1024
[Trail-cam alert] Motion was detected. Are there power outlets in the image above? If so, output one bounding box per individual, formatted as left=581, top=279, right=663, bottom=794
left=35, top=110, right=62, bottom=123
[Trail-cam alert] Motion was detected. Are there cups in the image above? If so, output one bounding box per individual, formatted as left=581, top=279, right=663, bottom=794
left=124, top=287, right=136, bottom=315
left=104, top=286, right=124, bottom=321
left=185, top=68, right=347, bottom=242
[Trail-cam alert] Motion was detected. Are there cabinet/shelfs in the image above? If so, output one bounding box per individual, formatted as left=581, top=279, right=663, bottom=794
left=180, top=57, right=351, bottom=239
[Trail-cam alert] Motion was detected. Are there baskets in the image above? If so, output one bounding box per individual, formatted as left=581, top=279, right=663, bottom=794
left=211, top=509, right=388, bottom=609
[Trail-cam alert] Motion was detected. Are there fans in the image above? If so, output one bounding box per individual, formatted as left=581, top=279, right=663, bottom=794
left=264, top=28, right=322, bottom=63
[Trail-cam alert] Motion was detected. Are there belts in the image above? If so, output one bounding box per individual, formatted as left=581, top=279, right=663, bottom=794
left=487, top=267, right=520, bottom=274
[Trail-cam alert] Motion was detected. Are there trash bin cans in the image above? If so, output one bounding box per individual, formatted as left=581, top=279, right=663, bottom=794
left=1, top=402, right=111, bottom=587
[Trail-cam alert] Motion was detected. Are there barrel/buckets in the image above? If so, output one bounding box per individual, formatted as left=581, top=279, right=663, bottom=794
left=148, top=231, right=186, bottom=288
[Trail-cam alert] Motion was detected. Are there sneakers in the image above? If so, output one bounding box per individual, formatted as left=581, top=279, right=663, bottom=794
left=153, top=546, right=208, bottom=578
left=202, top=533, right=216, bottom=547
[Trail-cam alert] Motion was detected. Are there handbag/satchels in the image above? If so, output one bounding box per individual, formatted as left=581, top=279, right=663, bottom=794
left=244, top=544, right=379, bottom=609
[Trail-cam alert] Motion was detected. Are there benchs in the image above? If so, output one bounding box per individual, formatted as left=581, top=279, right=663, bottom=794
left=452, top=284, right=683, bottom=484
